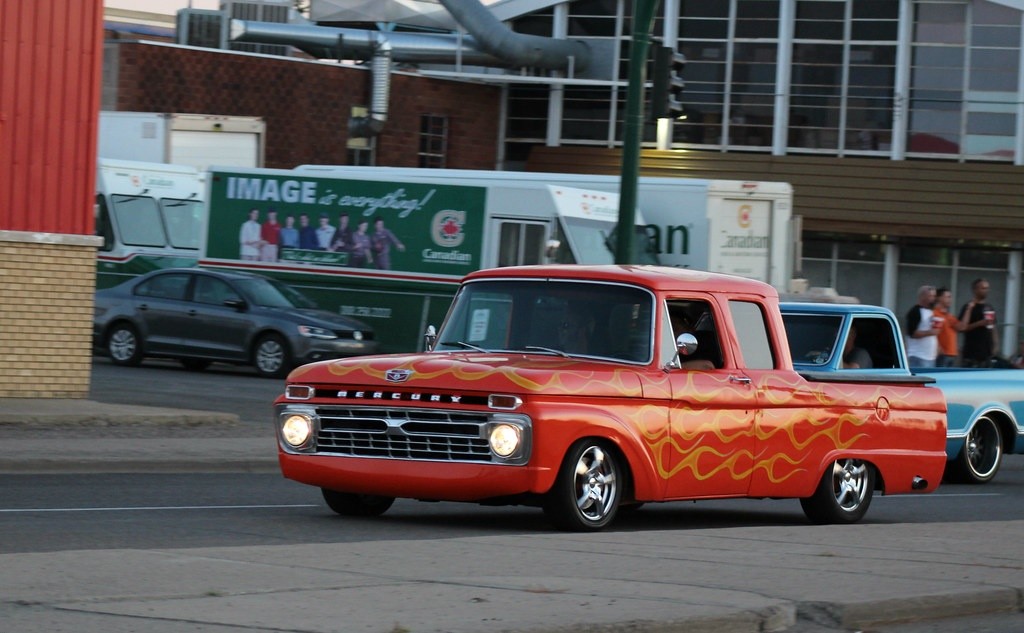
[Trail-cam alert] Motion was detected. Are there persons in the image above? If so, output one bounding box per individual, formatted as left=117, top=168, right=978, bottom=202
left=684, top=360, right=717, bottom=370
left=239, top=209, right=408, bottom=271
left=805, top=328, right=874, bottom=370
left=906, top=277, right=1000, bottom=368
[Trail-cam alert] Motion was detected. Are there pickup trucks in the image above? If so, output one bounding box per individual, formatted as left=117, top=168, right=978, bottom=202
left=777, top=302, right=1024, bottom=484
left=273, top=264, right=949, bottom=536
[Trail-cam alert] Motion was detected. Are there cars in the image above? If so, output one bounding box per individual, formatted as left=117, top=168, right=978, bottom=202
left=93, top=268, right=379, bottom=379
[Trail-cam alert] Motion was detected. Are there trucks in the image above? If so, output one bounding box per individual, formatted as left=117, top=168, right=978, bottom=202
left=94, top=113, right=797, bottom=356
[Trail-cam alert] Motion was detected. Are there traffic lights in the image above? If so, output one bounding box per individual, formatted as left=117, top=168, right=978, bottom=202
left=652, top=47, right=688, bottom=119
